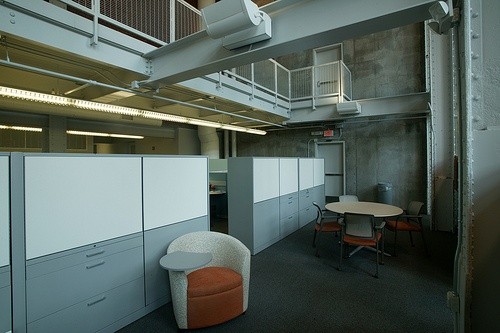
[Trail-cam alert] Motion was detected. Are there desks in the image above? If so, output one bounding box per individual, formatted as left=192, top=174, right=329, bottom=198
left=324, top=201, right=403, bottom=259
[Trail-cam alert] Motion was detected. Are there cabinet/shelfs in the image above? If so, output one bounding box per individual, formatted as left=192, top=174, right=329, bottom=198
left=227, top=158, right=325, bottom=256
left=0, top=151, right=210, bottom=333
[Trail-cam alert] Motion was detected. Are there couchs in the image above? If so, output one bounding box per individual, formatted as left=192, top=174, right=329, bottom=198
left=159, top=231, right=251, bottom=329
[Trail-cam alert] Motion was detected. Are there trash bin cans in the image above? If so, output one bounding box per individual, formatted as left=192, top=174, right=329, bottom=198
left=377, top=181, right=393, bottom=205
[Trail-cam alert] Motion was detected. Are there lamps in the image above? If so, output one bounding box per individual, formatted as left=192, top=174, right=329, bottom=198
left=200, top=0, right=272, bottom=50
left=427, top=0, right=456, bottom=35
left=0, top=83, right=267, bottom=136
left=336, top=101, right=361, bottom=115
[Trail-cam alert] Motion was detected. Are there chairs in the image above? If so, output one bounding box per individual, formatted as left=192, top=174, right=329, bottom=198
left=338, top=211, right=386, bottom=278
left=385, top=201, right=425, bottom=257
left=311, top=200, right=345, bottom=248
left=334, top=195, right=359, bottom=237
